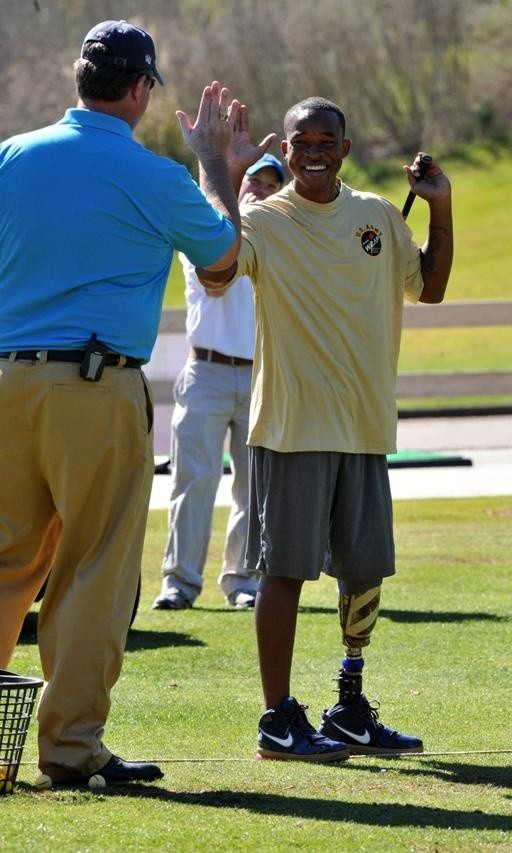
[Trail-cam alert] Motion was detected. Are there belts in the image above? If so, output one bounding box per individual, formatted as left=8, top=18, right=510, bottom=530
left=187, top=347, right=252, bottom=365
left=1, top=349, right=142, bottom=369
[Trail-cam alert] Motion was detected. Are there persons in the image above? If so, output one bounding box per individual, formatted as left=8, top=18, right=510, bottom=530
left=151, top=153, right=285, bottom=612
left=195, top=95, right=455, bottom=763
left=0, top=16, right=242, bottom=789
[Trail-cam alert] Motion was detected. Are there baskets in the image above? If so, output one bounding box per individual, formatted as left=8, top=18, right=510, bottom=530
left=0, top=675, right=43, bottom=796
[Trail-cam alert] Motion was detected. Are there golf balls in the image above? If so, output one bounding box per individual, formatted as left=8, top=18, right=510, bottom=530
left=0, top=762, right=14, bottom=794
left=86, top=776, right=106, bottom=788
left=35, top=776, right=51, bottom=790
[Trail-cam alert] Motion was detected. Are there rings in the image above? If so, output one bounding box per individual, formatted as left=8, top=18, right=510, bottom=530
left=220, top=113, right=227, bottom=120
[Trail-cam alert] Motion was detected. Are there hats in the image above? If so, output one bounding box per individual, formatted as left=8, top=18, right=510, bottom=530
left=82, top=19, right=167, bottom=85
left=247, top=153, right=287, bottom=184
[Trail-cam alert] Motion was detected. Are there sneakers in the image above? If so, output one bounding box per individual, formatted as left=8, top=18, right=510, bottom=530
left=318, top=700, right=424, bottom=754
left=257, top=698, right=350, bottom=761
left=153, top=591, right=192, bottom=610
left=225, top=587, right=256, bottom=607
left=44, top=753, right=165, bottom=786
